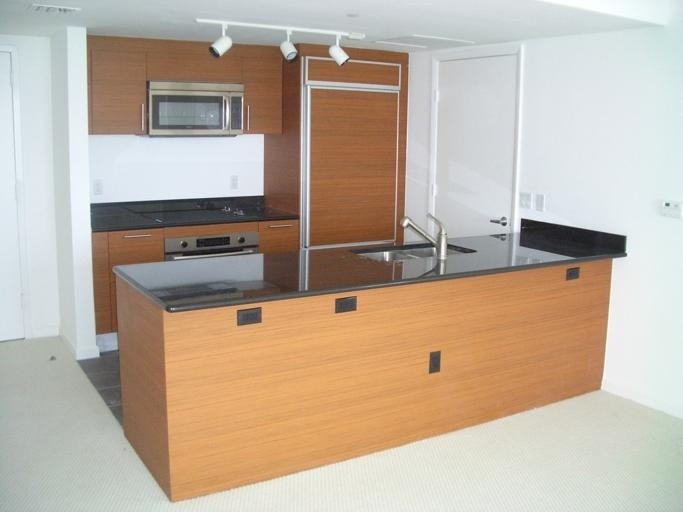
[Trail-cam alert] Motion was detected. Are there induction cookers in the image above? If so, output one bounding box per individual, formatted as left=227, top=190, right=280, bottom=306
left=137, top=202, right=256, bottom=227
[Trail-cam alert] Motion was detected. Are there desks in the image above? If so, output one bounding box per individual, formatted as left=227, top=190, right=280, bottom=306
left=108, top=217, right=630, bottom=503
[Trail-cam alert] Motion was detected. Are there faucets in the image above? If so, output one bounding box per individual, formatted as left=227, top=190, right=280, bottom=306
left=399, top=212, right=447, bottom=259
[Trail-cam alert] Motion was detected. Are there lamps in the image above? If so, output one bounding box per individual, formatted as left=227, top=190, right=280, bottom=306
left=189, top=13, right=355, bottom=69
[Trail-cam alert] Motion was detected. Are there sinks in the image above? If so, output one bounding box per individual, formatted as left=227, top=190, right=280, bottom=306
left=348, top=246, right=417, bottom=262
left=399, top=242, right=477, bottom=260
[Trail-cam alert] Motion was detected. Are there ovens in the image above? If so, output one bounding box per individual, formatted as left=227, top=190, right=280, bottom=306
left=164, top=247, right=260, bottom=261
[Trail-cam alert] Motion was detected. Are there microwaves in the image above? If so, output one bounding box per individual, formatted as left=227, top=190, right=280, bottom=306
left=146, top=80, right=244, bottom=139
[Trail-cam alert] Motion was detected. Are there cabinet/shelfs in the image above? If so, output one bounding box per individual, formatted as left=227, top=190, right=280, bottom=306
left=242, top=44, right=284, bottom=136
left=89, top=229, right=110, bottom=338
left=148, top=40, right=243, bottom=86
left=257, top=215, right=300, bottom=251
left=107, top=226, right=165, bottom=336
left=261, top=44, right=410, bottom=251
left=86, top=35, right=149, bottom=137
